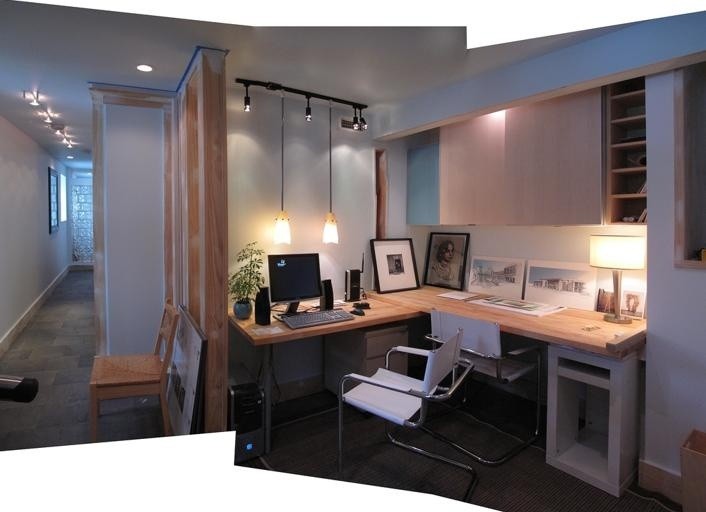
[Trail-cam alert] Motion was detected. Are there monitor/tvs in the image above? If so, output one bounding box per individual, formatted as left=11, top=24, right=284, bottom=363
left=268, top=253, right=323, bottom=322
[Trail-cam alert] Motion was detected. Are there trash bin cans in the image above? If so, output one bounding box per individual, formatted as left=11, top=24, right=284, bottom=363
left=680, top=429, right=706, bottom=512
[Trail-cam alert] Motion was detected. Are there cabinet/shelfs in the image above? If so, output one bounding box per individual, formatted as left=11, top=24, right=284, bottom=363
left=437, top=85, right=606, bottom=231
left=405, top=141, right=443, bottom=229
left=601, top=72, right=651, bottom=226
left=321, top=320, right=413, bottom=399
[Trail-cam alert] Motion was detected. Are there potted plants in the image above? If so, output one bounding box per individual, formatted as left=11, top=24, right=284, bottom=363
left=225, top=236, right=270, bottom=322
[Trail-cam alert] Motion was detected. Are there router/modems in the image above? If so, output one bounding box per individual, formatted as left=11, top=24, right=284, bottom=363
left=345, top=251, right=365, bottom=302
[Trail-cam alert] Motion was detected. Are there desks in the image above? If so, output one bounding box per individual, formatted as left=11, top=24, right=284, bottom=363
left=422, top=272, right=649, bottom=502
left=227, top=294, right=424, bottom=458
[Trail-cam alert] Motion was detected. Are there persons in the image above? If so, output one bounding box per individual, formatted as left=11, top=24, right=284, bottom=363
left=432, top=241, right=455, bottom=281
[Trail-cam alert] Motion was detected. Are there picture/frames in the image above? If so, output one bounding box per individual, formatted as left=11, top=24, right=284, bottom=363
left=420, top=230, right=473, bottom=295
left=367, top=235, right=424, bottom=297
left=466, top=254, right=528, bottom=302
left=523, top=257, right=598, bottom=313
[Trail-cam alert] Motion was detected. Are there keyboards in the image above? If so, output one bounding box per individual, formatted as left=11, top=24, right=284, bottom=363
left=283, top=307, right=354, bottom=330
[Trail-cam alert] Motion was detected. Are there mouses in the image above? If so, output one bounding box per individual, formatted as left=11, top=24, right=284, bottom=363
left=350, top=308, right=366, bottom=316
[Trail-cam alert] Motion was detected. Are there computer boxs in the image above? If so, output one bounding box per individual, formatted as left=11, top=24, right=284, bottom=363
left=226, top=362, right=265, bottom=464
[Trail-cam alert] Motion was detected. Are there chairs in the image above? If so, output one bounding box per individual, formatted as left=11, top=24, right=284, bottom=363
left=335, top=328, right=484, bottom=503
left=83, top=295, right=182, bottom=445
left=419, top=304, right=548, bottom=467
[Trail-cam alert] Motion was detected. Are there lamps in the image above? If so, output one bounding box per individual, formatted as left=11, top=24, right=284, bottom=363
left=584, top=232, right=648, bottom=328
left=232, top=74, right=375, bottom=249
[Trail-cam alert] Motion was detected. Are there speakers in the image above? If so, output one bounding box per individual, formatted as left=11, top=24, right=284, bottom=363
left=255, top=287, right=270, bottom=326
left=320, top=279, right=333, bottom=311
